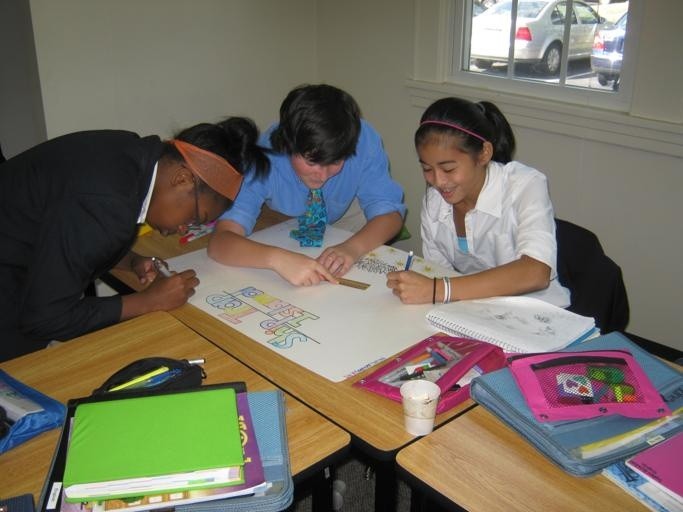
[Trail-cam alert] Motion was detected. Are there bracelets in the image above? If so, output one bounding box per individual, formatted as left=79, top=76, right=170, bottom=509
left=441, top=277, right=451, bottom=304
left=433, top=277, right=436, bottom=304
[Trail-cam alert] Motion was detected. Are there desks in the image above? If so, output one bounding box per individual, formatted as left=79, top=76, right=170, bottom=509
left=0, top=203, right=680, bottom=512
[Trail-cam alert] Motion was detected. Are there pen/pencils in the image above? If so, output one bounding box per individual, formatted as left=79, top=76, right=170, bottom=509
left=378, top=340, right=462, bottom=384
left=178, top=218, right=218, bottom=247
left=152, top=257, right=174, bottom=278
left=188, top=358, right=206, bottom=365
left=105, top=366, right=178, bottom=395
left=405, top=250, right=414, bottom=270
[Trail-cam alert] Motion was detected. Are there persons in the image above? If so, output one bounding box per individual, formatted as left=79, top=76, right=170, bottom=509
left=0, top=117, right=276, bottom=361
left=387, top=97, right=573, bottom=313
left=205, top=83, right=407, bottom=289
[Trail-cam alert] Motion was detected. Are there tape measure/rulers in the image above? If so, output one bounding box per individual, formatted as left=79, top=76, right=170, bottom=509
left=335, top=278, right=372, bottom=290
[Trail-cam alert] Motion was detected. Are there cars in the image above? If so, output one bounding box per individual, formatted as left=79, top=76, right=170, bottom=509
left=592, top=14, right=629, bottom=86
left=470, top=0, right=611, bottom=76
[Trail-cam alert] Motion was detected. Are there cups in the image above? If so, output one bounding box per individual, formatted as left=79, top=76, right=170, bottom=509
left=399, top=379, right=441, bottom=437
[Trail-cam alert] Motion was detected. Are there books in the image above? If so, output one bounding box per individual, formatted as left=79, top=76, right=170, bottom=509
left=426, top=295, right=601, bottom=353
left=41, top=380, right=267, bottom=512
left=602, top=431, right=683, bottom=512
